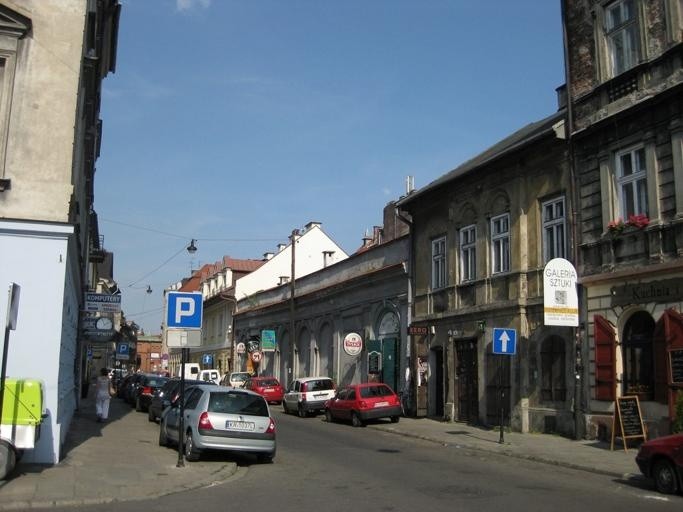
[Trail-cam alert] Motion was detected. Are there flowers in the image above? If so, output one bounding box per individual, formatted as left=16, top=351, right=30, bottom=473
left=604, top=213, right=650, bottom=240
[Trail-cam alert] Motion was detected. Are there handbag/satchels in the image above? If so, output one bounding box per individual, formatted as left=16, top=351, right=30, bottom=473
left=109, top=386, right=116, bottom=396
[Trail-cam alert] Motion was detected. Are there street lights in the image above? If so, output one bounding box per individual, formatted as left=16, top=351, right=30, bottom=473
left=286, top=228, right=299, bottom=388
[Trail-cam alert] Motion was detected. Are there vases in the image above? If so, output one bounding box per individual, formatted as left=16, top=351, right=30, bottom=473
left=609, top=225, right=647, bottom=240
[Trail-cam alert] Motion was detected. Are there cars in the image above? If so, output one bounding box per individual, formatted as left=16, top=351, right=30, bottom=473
left=107, top=367, right=170, bottom=413
left=239, top=377, right=284, bottom=405
left=157, top=384, right=277, bottom=462
left=323, top=382, right=402, bottom=428
left=147, top=379, right=216, bottom=424
left=635, top=433, right=682, bottom=495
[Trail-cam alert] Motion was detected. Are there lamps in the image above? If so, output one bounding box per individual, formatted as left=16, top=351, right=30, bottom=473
left=186, top=238, right=197, bottom=254
left=146, top=284, right=153, bottom=294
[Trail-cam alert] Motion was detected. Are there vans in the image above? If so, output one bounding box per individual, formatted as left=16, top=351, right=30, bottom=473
left=218, top=371, right=251, bottom=389
left=197, top=369, right=220, bottom=385
left=174, top=362, right=200, bottom=381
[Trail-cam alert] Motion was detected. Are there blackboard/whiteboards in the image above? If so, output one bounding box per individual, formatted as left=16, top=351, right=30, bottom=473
left=616, top=396, right=645, bottom=438
left=666, top=347, right=683, bottom=387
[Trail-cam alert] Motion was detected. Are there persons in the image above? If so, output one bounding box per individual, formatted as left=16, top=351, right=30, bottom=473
left=91, top=367, right=113, bottom=423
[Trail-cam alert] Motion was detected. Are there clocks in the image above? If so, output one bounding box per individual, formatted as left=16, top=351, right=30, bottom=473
left=96, top=316, right=114, bottom=332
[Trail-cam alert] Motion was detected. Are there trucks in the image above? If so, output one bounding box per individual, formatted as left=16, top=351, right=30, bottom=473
left=0, top=378, right=47, bottom=480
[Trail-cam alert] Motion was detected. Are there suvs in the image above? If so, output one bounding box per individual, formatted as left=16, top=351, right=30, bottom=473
left=282, top=376, right=337, bottom=417
